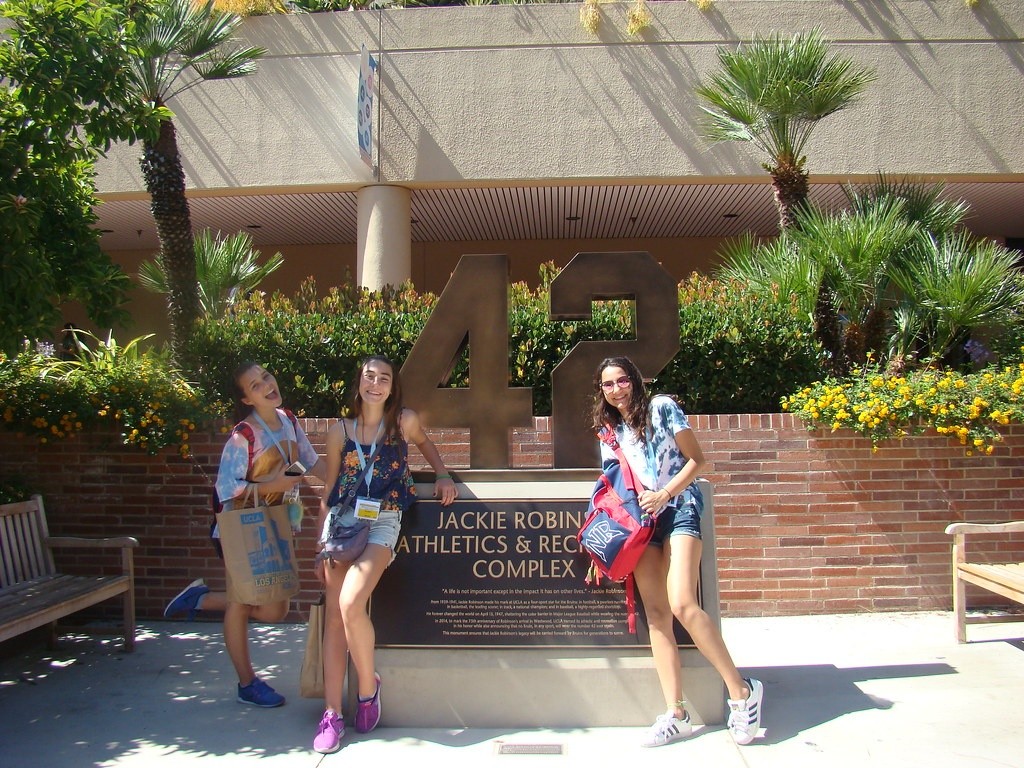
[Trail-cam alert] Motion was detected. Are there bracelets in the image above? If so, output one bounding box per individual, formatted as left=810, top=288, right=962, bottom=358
left=315, top=552, right=320, bottom=554
left=435, top=475, right=452, bottom=480
left=662, top=488, right=671, bottom=498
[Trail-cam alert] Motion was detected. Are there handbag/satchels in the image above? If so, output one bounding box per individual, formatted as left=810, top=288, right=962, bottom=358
left=216, top=483, right=300, bottom=606
left=326, top=519, right=371, bottom=563
left=301, top=582, right=326, bottom=698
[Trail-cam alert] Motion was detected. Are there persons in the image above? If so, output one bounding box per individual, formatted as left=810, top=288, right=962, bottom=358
left=162, top=362, right=325, bottom=707
left=312, top=356, right=458, bottom=754
left=591, top=356, right=764, bottom=748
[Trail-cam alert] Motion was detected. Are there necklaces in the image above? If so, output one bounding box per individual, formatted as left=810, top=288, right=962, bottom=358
left=360, top=411, right=382, bottom=444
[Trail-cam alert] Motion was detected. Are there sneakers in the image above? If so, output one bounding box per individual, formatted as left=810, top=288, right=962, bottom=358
left=164, top=578, right=209, bottom=623
left=354, top=672, right=381, bottom=733
left=238, top=677, right=285, bottom=707
left=313, top=706, right=345, bottom=754
left=642, top=710, right=692, bottom=747
left=727, top=677, right=763, bottom=745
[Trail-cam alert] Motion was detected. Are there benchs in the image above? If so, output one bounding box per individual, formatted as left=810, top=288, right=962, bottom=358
left=945, top=520, right=1024, bottom=644
left=1, top=494, right=140, bottom=654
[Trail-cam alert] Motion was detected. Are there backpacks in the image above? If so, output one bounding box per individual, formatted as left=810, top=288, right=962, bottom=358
left=577, top=423, right=656, bottom=582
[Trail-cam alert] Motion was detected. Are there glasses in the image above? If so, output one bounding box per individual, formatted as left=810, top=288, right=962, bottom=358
left=599, top=376, right=630, bottom=393
